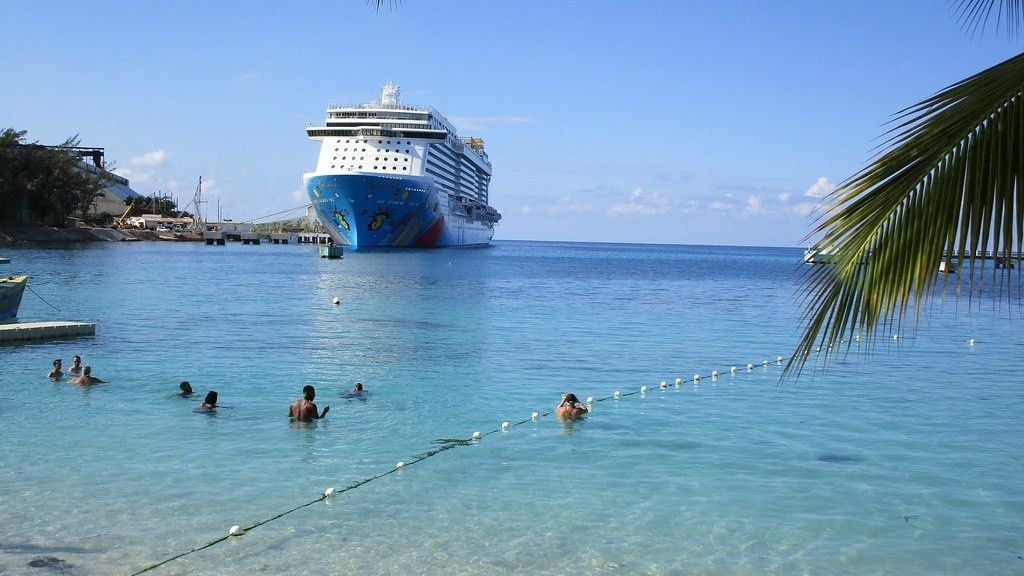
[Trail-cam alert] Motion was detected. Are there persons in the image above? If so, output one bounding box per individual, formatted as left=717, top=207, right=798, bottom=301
left=557, top=394, right=588, bottom=421
left=68, top=356, right=82, bottom=374
left=200, top=391, right=218, bottom=409
left=69, top=365, right=105, bottom=383
left=345, top=383, right=368, bottom=405
left=47, top=359, right=64, bottom=376
left=180, top=381, right=196, bottom=396
left=287, top=385, right=330, bottom=419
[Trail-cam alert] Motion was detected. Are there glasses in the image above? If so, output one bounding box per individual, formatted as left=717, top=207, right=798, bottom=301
left=74, top=361, right=80, bottom=363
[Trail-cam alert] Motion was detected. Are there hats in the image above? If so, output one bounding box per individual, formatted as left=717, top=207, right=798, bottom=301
left=566, top=394, right=576, bottom=404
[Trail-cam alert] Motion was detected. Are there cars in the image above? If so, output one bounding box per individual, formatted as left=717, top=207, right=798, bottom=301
left=157, top=225, right=170, bottom=232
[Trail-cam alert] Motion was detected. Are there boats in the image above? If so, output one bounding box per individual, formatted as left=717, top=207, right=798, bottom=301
left=940, top=260, right=957, bottom=273
left=802, top=241, right=872, bottom=265
left=0, top=274, right=29, bottom=322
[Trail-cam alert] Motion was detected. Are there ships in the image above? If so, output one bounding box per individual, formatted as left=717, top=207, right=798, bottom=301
left=302, top=82, right=502, bottom=248
